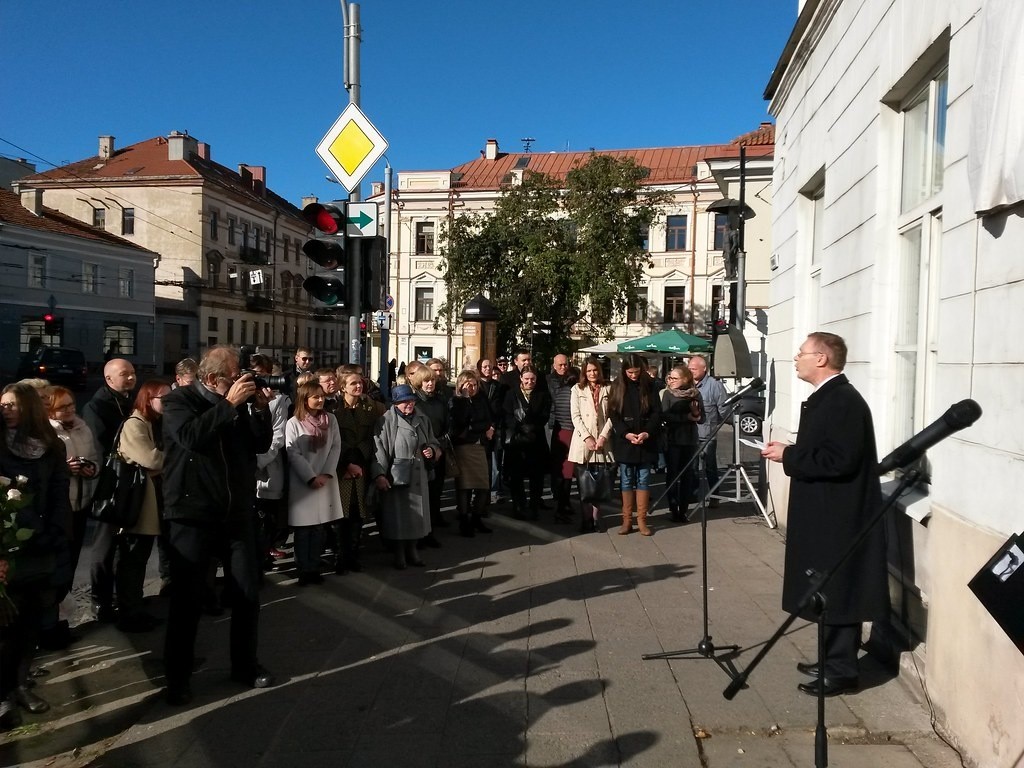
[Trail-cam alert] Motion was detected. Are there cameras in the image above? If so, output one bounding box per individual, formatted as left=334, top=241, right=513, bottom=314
left=73, top=456, right=90, bottom=470
left=239, top=369, right=293, bottom=395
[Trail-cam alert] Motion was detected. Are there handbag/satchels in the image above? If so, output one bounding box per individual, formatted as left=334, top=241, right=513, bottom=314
left=575, top=447, right=619, bottom=503
left=443, top=433, right=460, bottom=479
left=384, top=458, right=413, bottom=486
left=87, top=417, right=151, bottom=530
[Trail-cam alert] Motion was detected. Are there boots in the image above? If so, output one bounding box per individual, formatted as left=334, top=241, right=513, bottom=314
left=395, top=542, right=407, bottom=569
left=407, top=541, right=426, bottom=568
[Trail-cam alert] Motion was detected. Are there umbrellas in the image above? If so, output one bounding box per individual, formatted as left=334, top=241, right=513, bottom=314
left=618, top=329, right=714, bottom=369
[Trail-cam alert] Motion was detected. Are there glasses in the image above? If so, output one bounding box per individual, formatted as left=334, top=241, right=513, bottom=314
left=153, top=395, right=167, bottom=400
left=497, top=363, right=508, bottom=366
left=298, top=356, right=314, bottom=362
left=0, top=401, right=17, bottom=409
left=53, top=401, right=75, bottom=412
left=668, top=376, right=685, bottom=380
left=798, top=351, right=829, bottom=360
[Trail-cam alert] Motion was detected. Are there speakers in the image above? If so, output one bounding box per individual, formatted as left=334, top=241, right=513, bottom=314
left=714, top=326, right=753, bottom=377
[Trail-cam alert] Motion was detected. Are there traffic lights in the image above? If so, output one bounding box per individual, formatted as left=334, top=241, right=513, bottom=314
left=359, top=320, right=367, bottom=345
left=43, top=313, right=56, bottom=336
left=300, top=201, right=350, bottom=315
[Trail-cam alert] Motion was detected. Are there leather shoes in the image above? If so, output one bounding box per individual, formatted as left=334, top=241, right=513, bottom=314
left=798, top=675, right=854, bottom=696
left=797, top=662, right=819, bottom=676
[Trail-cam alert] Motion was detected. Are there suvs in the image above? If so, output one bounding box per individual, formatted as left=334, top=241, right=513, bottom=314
left=16, top=342, right=89, bottom=394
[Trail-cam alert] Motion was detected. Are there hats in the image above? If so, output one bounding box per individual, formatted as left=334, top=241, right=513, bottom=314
left=497, top=356, right=510, bottom=363
left=389, top=385, right=418, bottom=404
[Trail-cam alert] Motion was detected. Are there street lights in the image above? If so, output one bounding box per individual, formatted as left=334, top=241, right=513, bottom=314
left=705, top=138, right=757, bottom=327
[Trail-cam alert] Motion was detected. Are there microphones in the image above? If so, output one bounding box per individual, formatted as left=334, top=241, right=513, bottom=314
left=721, top=377, right=763, bottom=407
left=874, top=398, right=983, bottom=476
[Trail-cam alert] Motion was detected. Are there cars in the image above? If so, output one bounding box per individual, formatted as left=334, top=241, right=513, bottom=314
left=722, top=383, right=766, bottom=436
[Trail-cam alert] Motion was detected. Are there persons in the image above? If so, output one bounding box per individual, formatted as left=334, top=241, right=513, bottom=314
left=0, top=344, right=733, bottom=732
left=762, top=333, right=892, bottom=696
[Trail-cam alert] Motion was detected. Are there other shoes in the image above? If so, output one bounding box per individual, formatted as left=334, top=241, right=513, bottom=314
left=0, top=699, right=22, bottom=729
left=9, top=686, right=51, bottom=714
left=265, top=532, right=371, bottom=587
left=24, top=664, right=50, bottom=677
left=238, top=663, right=273, bottom=687
left=114, top=612, right=161, bottom=633
left=420, top=477, right=719, bottom=549
left=166, top=674, right=192, bottom=705
left=20, top=672, right=37, bottom=689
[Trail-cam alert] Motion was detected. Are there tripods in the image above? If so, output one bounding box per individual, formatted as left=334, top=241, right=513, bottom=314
left=686, top=377, right=774, bottom=529
left=641, top=393, right=748, bottom=688
